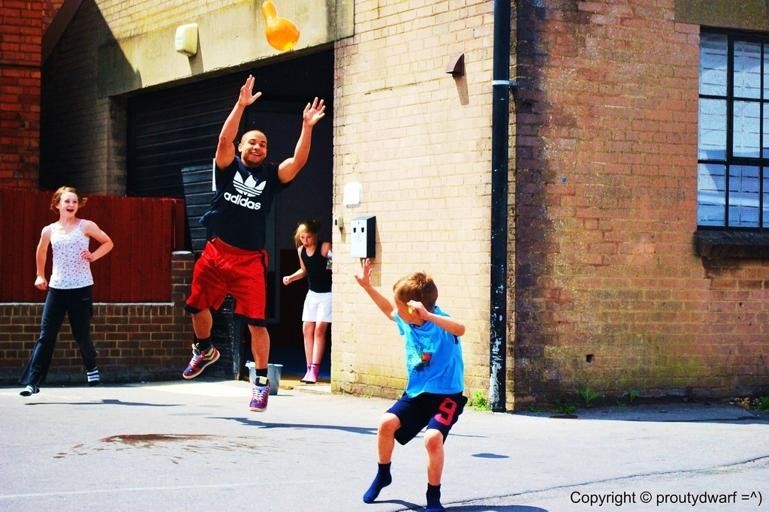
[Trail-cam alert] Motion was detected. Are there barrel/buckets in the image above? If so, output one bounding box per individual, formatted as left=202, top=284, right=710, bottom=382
left=244, top=362, right=284, bottom=395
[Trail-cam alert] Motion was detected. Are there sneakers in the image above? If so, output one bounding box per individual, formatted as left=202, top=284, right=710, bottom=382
left=249, top=380, right=271, bottom=412
left=183, top=344, right=220, bottom=379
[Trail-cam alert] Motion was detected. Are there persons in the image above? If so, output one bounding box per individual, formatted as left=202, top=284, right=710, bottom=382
left=17, top=185, right=113, bottom=397
left=179, top=75, right=327, bottom=413
left=282, top=220, right=334, bottom=384
left=353, top=258, right=468, bottom=511
